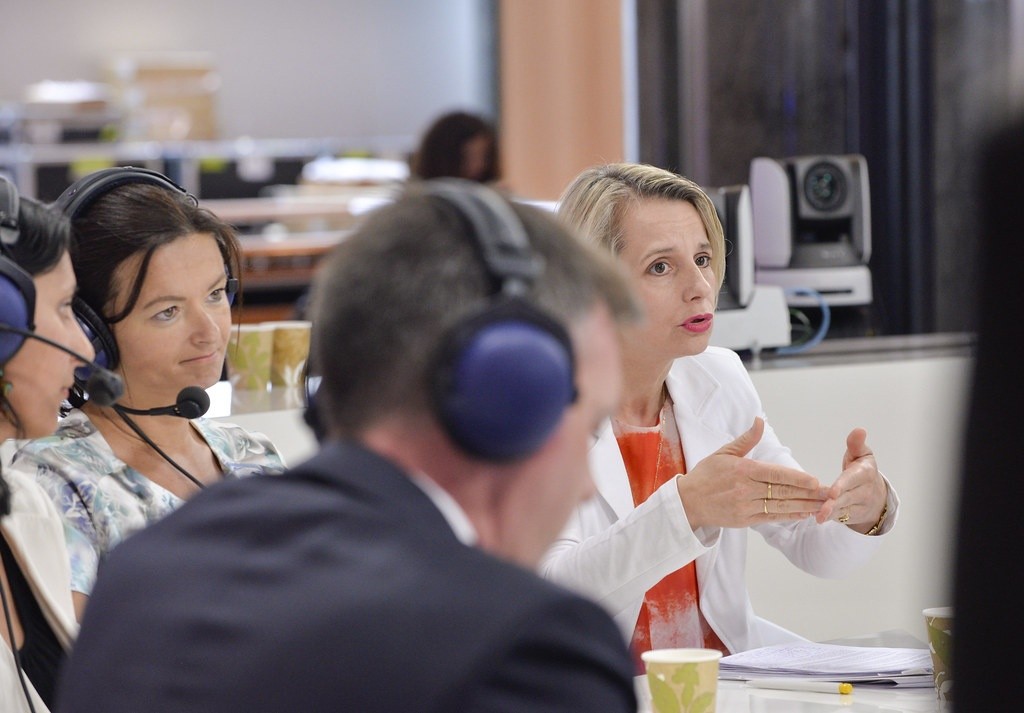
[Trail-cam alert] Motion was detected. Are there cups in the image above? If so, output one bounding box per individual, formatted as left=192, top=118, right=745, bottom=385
left=922, top=606, right=956, bottom=713
left=640, top=647, right=723, bottom=713
left=226, top=323, right=278, bottom=391
left=259, top=319, right=313, bottom=386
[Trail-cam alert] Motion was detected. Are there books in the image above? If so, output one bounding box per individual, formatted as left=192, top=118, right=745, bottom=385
left=717, top=643, right=933, bottom=687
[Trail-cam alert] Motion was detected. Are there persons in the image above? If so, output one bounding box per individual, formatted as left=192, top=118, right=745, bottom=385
left=535, top=162, right=900, bottom=676
left=0, top=177, right=95, bottom=713
left=15, top=167, right=286, bottom=629
left=49, top=179, right=637, bottom=713
left=415, top=114, right=497, bottom=180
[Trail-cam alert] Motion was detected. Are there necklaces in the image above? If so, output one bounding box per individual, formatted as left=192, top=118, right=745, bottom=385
left=633, top=385, right=666, bottom=506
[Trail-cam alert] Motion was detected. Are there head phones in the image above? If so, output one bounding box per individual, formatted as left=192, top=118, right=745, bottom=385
left=298, top=176, right=581, bottom=463
left=0, top=174, right=36, bottom=363
left=54, top=163, right=240, bottom=386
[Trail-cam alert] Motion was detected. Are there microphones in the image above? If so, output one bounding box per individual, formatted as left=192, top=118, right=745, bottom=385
left=110, top=386, right=212, bottom=421
left=0, top=322, right=124, bottom=406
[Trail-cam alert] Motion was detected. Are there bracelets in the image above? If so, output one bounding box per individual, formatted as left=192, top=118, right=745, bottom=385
left=867, top=506, right=888, bottom=534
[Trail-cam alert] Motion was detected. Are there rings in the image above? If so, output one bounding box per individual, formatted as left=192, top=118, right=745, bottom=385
left=768, top=483, right=771, bottom=500
left=765, top=500, right=768, bottom=513
left=840, top=515, right=849, bottom=523
